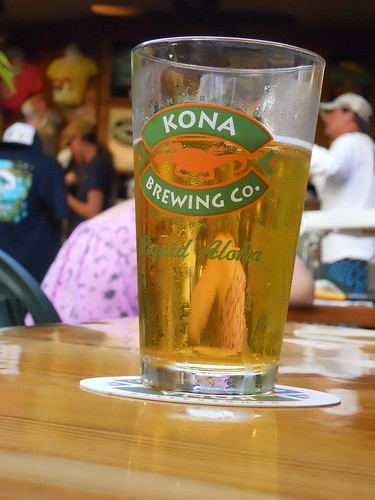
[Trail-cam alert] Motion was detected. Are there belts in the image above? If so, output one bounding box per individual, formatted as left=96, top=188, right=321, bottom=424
left=324, top=228, right=375, bottom=237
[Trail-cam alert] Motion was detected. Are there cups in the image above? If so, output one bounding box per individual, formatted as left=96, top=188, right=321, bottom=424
left=131, top=35, right=326, bottom=394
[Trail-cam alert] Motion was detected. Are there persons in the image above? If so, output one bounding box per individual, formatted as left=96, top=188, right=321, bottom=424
left=67, top=128, right=115, bottom=237
left=24, top=198, right=315, bottom=327
left=308, top=93, right=375, bottom=294
left=0, top=107, right=69, bottom=283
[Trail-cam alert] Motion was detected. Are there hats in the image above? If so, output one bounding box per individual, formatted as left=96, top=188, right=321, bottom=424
left=319, top=91, right=373, bottom=124
left=58, top=108, right=97, bottom=148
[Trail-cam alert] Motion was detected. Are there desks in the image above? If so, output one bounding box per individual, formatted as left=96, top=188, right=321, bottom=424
left=0, top=317, right=374, bottom=500
